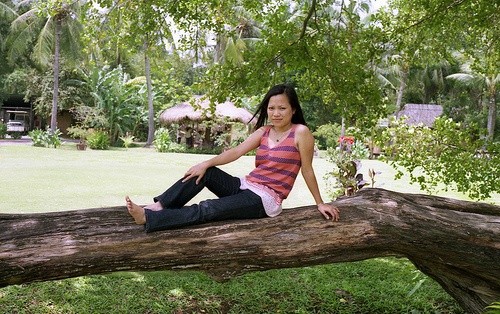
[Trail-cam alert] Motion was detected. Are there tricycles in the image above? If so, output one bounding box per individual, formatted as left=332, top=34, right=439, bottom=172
left=6, top=110, right=31, bottom=136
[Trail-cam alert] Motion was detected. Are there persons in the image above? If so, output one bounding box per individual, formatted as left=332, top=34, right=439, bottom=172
left=176, top=128, right=185, bottom=144
left=313, top=143, right=320, bottom=153
left=124, top=84, right=340, bottom=235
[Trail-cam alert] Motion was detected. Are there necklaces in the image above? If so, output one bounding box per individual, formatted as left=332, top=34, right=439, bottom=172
left=272, top=126, right=291, bottom=142
